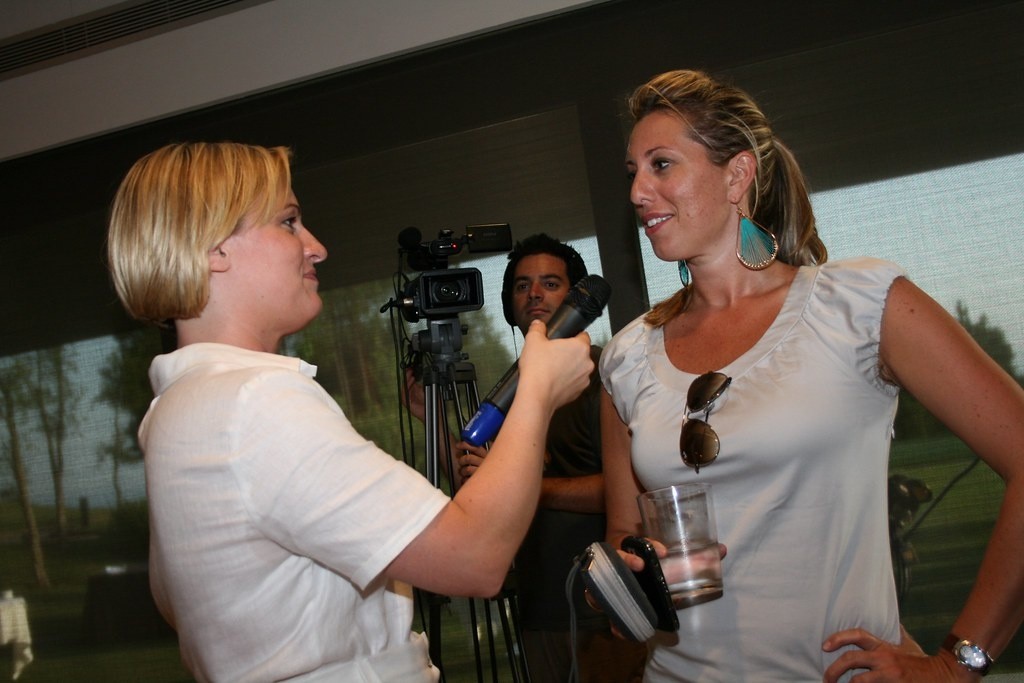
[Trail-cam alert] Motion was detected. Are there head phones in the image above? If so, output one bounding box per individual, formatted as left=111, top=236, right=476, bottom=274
left=501, top=240, right=588, bottom=324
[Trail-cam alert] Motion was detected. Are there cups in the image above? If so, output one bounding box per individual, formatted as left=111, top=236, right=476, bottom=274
left=638, top=481, right=724, bottom=608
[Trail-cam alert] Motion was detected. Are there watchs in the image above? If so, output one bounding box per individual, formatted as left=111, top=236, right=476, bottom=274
left=940, top=633, right=995, bottom=677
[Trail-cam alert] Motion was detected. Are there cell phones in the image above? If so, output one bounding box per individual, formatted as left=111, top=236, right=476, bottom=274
left=621, top=535, right=680, bottom=633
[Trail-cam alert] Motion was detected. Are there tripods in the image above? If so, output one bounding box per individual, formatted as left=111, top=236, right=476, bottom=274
left=406, top=319, right=537, bottom=683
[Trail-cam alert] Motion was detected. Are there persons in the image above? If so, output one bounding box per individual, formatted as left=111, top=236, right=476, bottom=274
left=108, top=142, right=595, bottom=683
left=400, top=233, right=647, bottom=683
left=599, top=69, right=1024, bottom=683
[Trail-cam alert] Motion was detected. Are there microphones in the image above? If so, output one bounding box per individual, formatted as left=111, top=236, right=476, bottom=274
left=398, top=226, right=422, bottom=250
left=461, top=275, right=612, bottom=447
